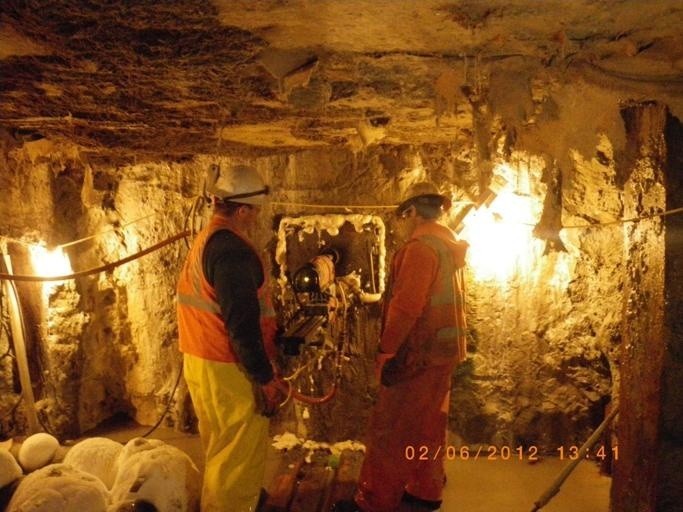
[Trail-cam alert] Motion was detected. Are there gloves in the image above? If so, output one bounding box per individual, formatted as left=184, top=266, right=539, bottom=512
left=374, top=352, right=395, bottom=385
left=256, top=382, right=281, bottom=418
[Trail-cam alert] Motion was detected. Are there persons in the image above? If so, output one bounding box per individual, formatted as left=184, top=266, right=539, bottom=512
left=333, top=181, right=469, bottom=512
left=177, top=165, right=289, bottom=511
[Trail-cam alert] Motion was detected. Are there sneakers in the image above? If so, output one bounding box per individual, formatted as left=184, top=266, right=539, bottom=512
left=401, top=491, right=442, bottom=512
left=330, top=499, right=363, bottom=512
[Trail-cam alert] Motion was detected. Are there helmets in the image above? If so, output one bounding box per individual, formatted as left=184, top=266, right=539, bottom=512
left=214, top=166, right=267, bottom=206
left=395, top=183, right=451, bottom=216
